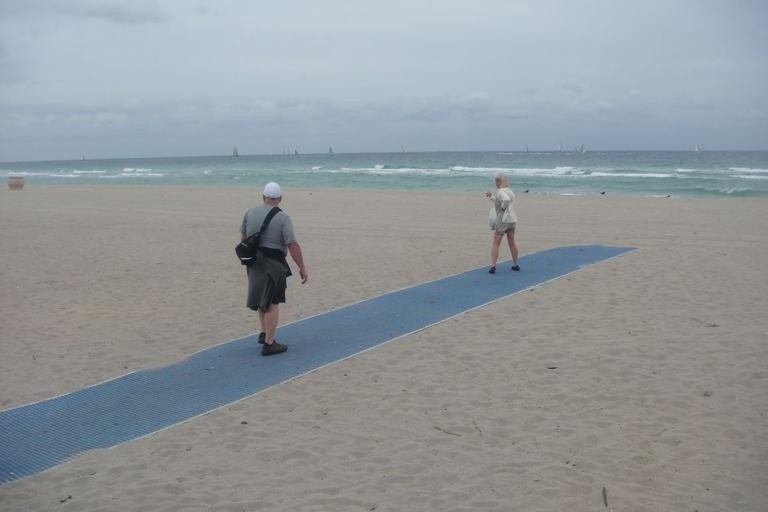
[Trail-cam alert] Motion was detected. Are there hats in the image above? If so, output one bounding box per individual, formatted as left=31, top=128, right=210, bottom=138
left=263, top=182, right=281, bottom=199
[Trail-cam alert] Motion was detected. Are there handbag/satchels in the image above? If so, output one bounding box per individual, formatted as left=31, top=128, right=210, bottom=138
left=235, top=231, right=259, bottom=265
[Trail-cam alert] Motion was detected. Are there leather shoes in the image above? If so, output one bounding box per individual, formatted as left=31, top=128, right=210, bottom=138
left=512, top=266, right=520, bottom=271
left=489, top=266, right=496, bottom=273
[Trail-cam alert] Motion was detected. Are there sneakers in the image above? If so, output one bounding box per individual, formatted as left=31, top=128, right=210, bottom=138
left=258, top=332, right=288, bottom=355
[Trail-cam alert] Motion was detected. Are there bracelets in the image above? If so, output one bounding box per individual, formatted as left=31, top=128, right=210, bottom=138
left=501, top=208, right=506, bottom=211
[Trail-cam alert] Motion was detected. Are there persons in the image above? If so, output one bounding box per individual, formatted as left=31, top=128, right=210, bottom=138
left=239, top=181, right=307, bottom=356
left=486, top=174, right=521, bottom=273
